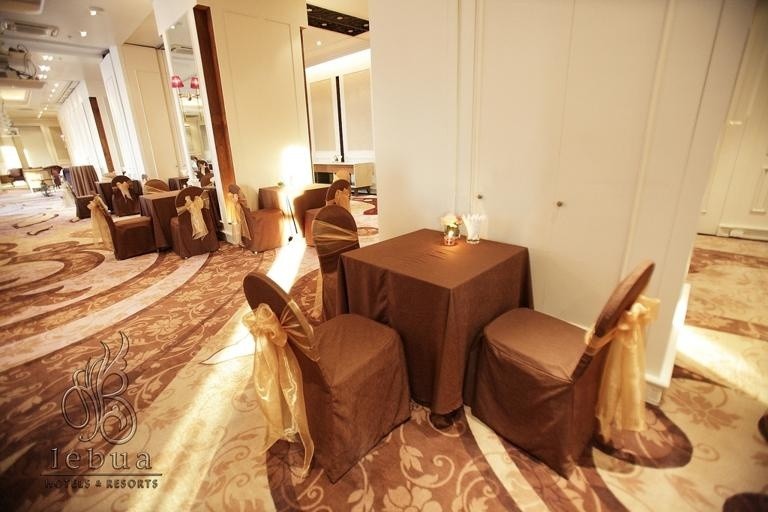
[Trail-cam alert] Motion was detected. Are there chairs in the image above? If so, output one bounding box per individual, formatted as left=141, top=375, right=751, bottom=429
left=0, top=166, right=655, bottom=484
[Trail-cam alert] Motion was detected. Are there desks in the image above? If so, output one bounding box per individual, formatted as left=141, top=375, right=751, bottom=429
left=313, top=163, right=373, bottom=197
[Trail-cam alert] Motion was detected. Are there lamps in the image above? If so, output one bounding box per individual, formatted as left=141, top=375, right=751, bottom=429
left=170, top=75, right=188, bottom=101
left=189, top=75, right=200, bottom=100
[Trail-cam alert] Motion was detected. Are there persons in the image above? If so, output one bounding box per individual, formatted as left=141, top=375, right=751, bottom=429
left=186, top=154, right=198, bottom=172
